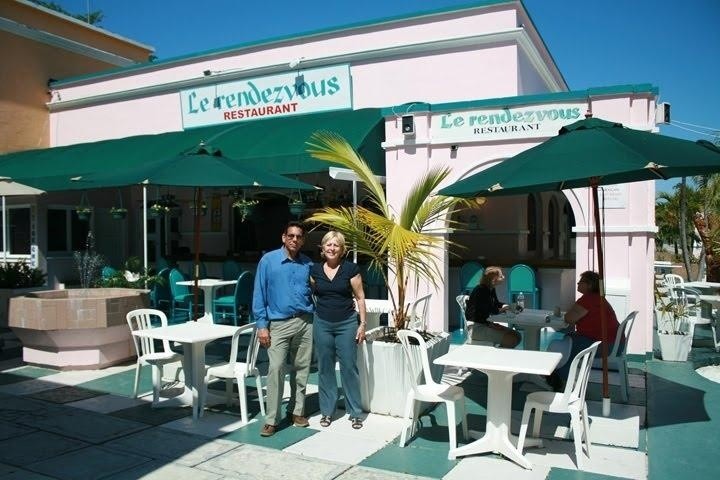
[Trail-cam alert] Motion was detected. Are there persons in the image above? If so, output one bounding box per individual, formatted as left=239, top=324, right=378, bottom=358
left=466, top=266, right=522, bottom=350
left=554, top=271, right=627, bottom=393
left=309, top=230, right=369, bottom=430
left=251, top=223, right=316, bottom=437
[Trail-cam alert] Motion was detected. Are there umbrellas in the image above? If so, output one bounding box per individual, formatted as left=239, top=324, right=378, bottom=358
left=435, top=115, right=720, bottom=417
left=0, top=176, right=50, bottom=268
left=67, top=144, right=324, bottom=322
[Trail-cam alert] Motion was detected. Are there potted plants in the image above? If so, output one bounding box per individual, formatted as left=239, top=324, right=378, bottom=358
left=300, top=125, right=486, bottom=423
left=0, top=258, right=50, bottom=330
left=72, top=197, right=308, bottom=222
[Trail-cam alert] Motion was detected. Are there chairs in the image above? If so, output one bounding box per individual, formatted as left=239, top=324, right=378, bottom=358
left=363, top=261, right=389, bottom=300
left=95, top=253, right=275, bottom=427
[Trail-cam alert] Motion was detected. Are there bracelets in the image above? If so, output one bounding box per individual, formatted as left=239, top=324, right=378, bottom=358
left=359, top=320, right=368, bottom=326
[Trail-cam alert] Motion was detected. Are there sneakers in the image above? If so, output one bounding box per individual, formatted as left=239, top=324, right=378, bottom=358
left=352, top=418, right=362, bottom=429
left=320, top=416, right=331, bottom=427
left=260, top=424, right=279, bottom=436
left=287, top=413, right=309, bottom=427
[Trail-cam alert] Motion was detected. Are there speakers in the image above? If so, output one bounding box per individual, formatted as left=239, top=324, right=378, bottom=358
left=656, top=101, right=671, bottom=125
left=401, top=114, right=415, bottom=135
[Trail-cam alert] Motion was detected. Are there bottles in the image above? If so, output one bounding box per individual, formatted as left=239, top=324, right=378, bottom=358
left=517, top=292, right=525, bottom=309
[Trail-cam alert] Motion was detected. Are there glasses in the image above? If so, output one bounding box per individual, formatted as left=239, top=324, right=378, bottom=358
left=285, top=234, right=302, bottom=239
